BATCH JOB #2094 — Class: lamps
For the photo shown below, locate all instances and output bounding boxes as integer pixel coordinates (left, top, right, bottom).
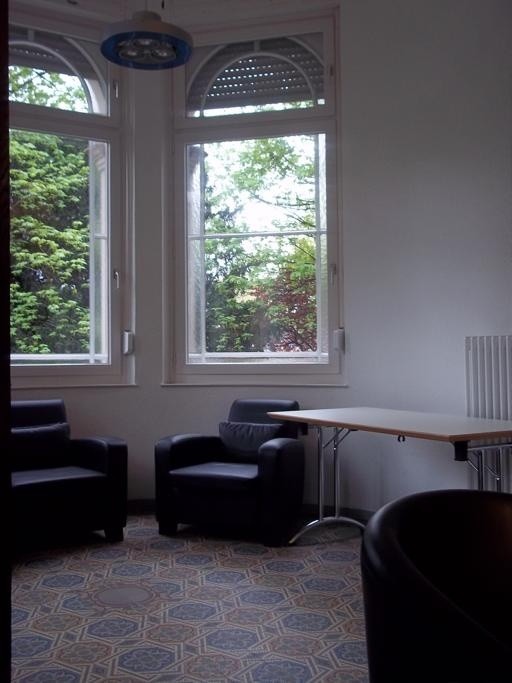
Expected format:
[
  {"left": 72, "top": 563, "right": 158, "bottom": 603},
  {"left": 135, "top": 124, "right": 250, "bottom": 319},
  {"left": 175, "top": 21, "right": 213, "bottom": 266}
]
[{"left": 100, "top": 2, "right": 193, "bottom": 70}]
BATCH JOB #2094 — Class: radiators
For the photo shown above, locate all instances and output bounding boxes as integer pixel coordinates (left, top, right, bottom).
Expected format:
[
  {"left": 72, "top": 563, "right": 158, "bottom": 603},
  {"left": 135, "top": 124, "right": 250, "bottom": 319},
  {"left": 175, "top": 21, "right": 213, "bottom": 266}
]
[{"left": 464, "top": 336, "right": 512, "bottom": 492}]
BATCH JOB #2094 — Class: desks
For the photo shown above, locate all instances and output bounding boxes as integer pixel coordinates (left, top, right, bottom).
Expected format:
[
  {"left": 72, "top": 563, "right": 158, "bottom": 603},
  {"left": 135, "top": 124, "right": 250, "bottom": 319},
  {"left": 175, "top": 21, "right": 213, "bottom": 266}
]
[{"left": 264, "top": 407, "right": 512, "bottom": 544}]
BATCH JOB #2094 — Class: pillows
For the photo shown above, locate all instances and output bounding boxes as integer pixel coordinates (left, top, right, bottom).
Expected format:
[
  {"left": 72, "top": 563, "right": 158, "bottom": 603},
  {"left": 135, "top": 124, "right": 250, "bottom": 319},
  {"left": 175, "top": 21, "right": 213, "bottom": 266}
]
[
  {"left": 10, "top": 421, "right": 70, "bottom": 468},
  {"left": 218, "top": 421, "right": 283, "bottom": 464}
]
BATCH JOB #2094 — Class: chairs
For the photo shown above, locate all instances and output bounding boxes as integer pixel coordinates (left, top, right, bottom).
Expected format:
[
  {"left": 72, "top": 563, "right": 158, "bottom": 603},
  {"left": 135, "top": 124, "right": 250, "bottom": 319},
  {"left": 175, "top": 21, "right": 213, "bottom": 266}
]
[
  {"left": 361, "top": 489, "right": 512, "bottom": 683},
  {"left": 154, "top": 398, "right": 306, "bottom": 548},
  {"left": 9, "top": 399, "right": 128, "bottom": 563}
]
